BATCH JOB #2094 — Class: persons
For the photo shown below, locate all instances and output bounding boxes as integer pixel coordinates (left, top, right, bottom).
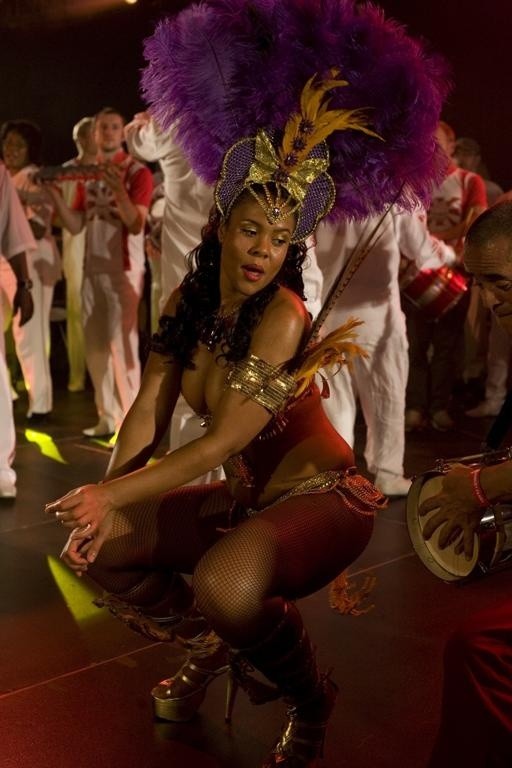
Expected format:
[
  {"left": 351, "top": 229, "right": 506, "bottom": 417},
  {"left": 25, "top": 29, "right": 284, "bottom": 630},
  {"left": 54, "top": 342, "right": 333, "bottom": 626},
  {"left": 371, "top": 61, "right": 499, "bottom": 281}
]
[
  {"left": 425, "top": 194, "right": 512, "bottom": 767},
  {"left": 1, "top": 100, "right": 512, "bottom": 511},
  {"left": 40, "top": 172, "right": 393, "bottom": 768}
]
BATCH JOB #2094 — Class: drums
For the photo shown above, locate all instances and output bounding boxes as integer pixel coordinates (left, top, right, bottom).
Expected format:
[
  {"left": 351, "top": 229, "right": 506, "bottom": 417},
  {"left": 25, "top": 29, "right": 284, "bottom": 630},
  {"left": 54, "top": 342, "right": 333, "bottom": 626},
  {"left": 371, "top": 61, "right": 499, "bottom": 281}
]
[
  {"left": 405, "top": 443, "right": 509, "bottom": 583},
  {"left": 395, "top": 239, "right": 470, "bottom": 324}
]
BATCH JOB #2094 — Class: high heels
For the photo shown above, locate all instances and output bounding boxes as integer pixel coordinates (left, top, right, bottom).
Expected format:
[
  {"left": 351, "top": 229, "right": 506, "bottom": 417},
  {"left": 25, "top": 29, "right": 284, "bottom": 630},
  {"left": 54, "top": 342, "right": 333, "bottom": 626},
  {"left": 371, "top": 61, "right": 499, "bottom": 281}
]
[
  {"left": 151, "top": 644, "right": 237, "bottom": 722},
  {"left": 272, "top": 682, "right": 329, "bottom": 764}
]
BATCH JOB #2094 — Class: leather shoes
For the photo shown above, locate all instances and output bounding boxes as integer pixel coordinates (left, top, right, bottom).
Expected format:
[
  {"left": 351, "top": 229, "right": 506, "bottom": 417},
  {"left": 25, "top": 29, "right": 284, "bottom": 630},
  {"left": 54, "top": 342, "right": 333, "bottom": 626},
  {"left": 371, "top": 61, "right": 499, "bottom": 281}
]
[
  {"left": 69, "top": 380, "right": 85, "bottom": 391},
  {"left": 27, "top": 402, "right": 47, "bottom": 417},
  {"left": 83, "top": 422, "right": 114, "bottom": 436},
  {"left": 375, "top": 476, "right": 412, "bottom": 495},
  {"left": 1, "top": 485, "right": 18, "bottom": 498}
]
